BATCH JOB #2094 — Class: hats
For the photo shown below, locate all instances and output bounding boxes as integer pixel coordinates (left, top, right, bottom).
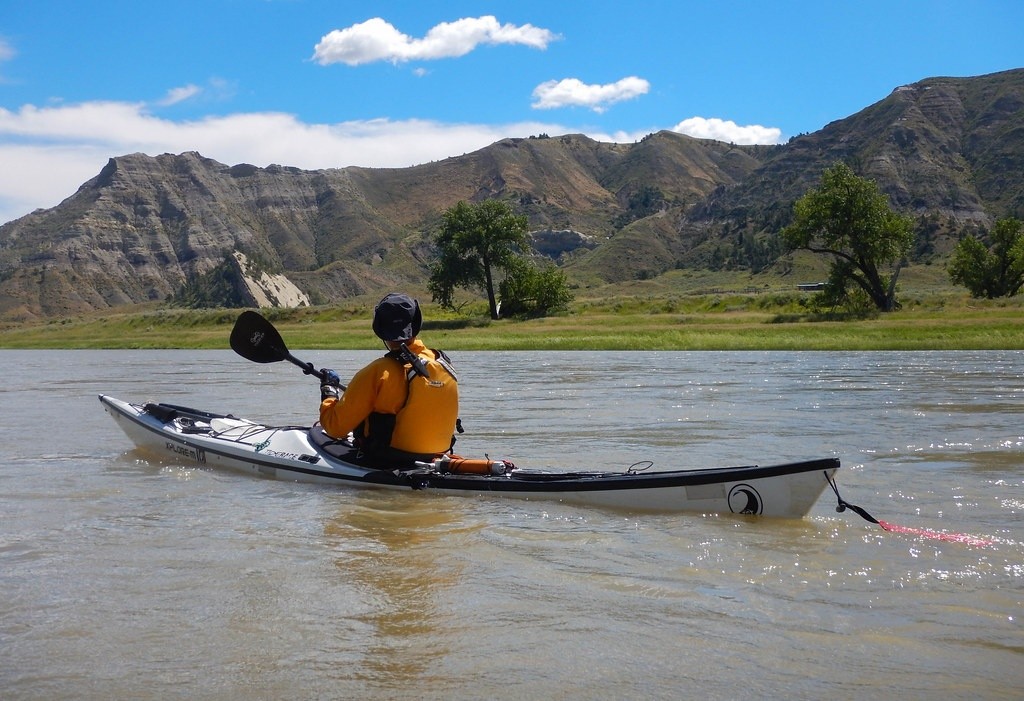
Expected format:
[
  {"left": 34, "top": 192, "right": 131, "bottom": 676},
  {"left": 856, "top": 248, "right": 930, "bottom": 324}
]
[{"left": 372, "top": 293, "right": 422, "bottom": 341}]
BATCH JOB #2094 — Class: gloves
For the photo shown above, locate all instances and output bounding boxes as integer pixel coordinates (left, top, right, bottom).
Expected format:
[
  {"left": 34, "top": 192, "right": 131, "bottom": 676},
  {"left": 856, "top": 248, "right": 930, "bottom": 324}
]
[{"left": 318, "top": 368, "right": 340, "bottom": 394}]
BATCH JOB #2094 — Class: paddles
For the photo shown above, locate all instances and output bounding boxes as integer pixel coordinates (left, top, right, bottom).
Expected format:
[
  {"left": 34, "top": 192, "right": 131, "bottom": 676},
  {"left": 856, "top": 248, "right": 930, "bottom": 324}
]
[{"left": 228, "top": 310, "right": 347, "bottom": 392}]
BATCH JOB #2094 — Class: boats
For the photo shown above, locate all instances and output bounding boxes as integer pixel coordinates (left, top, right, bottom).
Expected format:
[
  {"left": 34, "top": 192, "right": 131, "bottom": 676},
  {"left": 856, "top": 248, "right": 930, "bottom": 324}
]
[{"left": 97, "top": 391, "right": 840, "bottom": 522}]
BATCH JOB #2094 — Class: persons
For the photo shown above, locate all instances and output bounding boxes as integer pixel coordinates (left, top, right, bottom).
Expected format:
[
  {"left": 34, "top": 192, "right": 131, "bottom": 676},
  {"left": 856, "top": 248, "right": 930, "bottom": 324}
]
[{"left": 319, "top": 292, "right": 459, "bottom": 470}]
